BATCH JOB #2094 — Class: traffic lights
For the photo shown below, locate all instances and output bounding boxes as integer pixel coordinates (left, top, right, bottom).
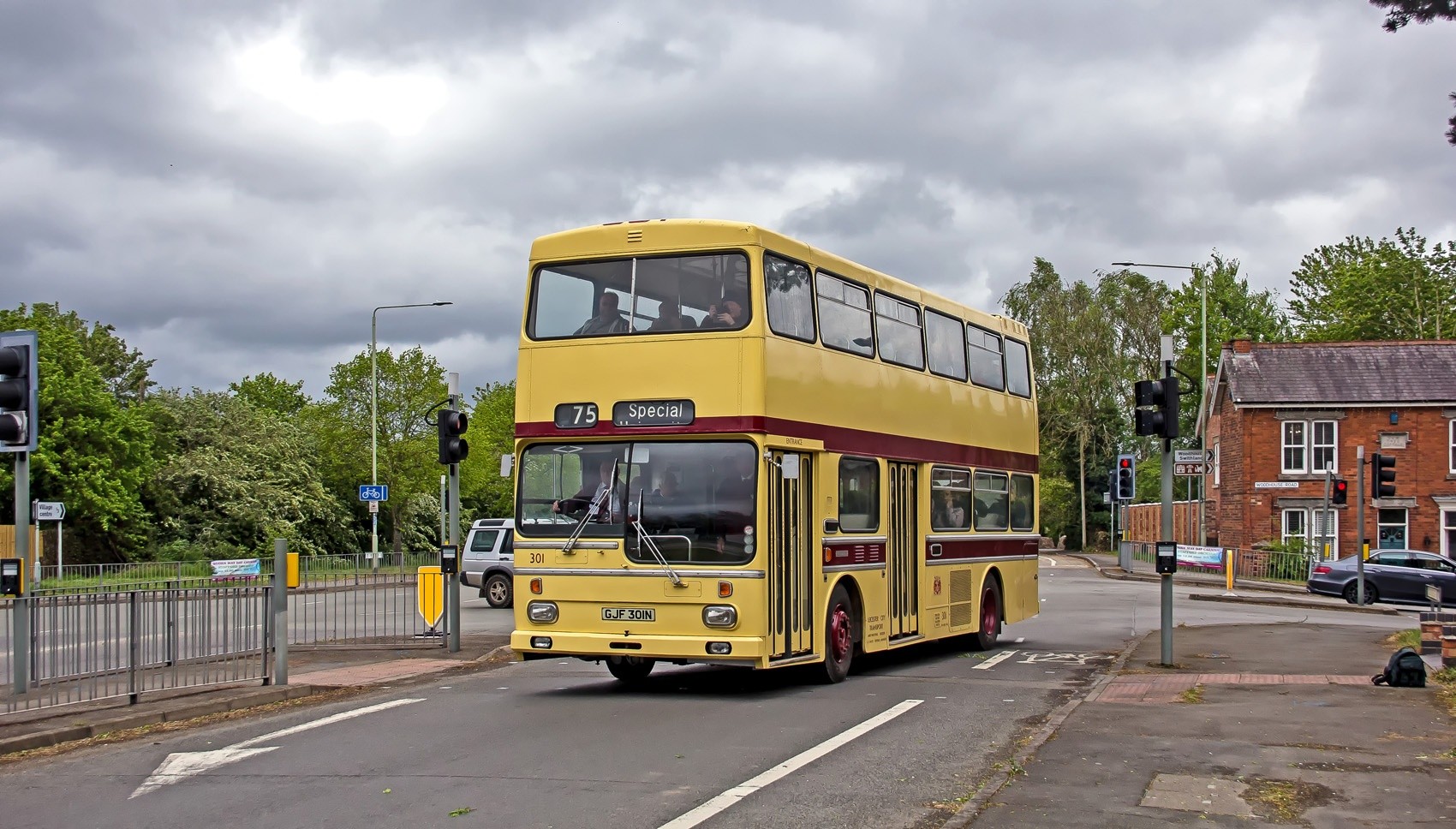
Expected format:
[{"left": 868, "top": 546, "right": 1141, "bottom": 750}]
[
  {"left": 1118, "top": 454, "right": 1135, "bottom": 499},
  {"left": 438, "top": 408, "right": 469, "bottom": 466},
  {"left": 1333, "top": 479, "right": 1347, "bottom": 504},
  {"left": 0, "top": 330, "right": 38, "bottom": 453},
  {"left": 1371, "top": 453, "right": 1396, "bottom": 500}
]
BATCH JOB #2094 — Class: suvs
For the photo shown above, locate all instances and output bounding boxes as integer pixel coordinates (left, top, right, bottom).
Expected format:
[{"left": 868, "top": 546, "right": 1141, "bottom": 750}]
[{"left": 459, "top": 518, "right": 572, "bottom": 609}]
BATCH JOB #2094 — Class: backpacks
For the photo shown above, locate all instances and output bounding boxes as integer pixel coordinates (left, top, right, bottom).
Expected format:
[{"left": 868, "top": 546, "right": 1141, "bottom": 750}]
[{"left": 1372, "top": 646, "right": 1428, "bottom": 688}]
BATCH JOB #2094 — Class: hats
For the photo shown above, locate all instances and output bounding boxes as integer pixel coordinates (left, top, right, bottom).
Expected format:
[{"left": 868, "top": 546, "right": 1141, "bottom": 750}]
[{"left": 722, "top": 289, "right": 745, "bottom": 309}]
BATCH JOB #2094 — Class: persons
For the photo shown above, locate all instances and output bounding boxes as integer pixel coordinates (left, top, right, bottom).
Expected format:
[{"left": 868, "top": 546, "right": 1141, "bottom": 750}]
[
  {"left": 931, "top": 479, "right": 1027, "bottom": 528},
  {"left": 629, "top": 472, "right": 700, "bottom": 529},
  {"left": 553, "top": 458, "right": 627, "bottom": 524},
  {"left": 714, "top": 451, "right": 755, "bottom": 554},
  {"left": 572, "top": 290, "right": 750, "bottom": 337}
]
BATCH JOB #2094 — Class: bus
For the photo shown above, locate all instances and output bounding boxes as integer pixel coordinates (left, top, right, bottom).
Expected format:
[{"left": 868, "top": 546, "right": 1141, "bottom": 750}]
[{"left": 498, "top": 217, "right": 1038, "bottom": 687}]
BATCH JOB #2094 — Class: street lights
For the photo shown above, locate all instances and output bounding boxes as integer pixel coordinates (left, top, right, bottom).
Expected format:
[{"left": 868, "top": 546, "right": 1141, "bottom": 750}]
[
  {"left": 370, "top": 301, "right": 454, "bottom": 575},
  {"left": 1112, "top": 260, "right": 1207, "bottom": 547}
]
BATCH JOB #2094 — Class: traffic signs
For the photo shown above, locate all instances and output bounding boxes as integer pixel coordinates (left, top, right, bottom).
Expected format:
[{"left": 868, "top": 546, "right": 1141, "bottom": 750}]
[
  {"left": 1174, "top": 462, "right": 1214, "bottom": 475},
  {"left": 32, "top": 500, "right": 66, "bottom": 520},
  {"left": 1174, "top": 449, "right": 1215, "bottom": 463}
]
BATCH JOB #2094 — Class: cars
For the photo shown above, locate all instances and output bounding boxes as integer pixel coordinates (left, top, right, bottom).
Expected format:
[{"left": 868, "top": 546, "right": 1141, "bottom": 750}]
[{"left": 1306, "top": 548, "right": 1456, "bottom": 609}]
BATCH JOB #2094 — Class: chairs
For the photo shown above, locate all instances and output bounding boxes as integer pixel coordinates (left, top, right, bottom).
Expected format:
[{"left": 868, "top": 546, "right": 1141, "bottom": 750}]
[{"left": 781, "top": 322, "right": 1017, "bottom": 393}]
